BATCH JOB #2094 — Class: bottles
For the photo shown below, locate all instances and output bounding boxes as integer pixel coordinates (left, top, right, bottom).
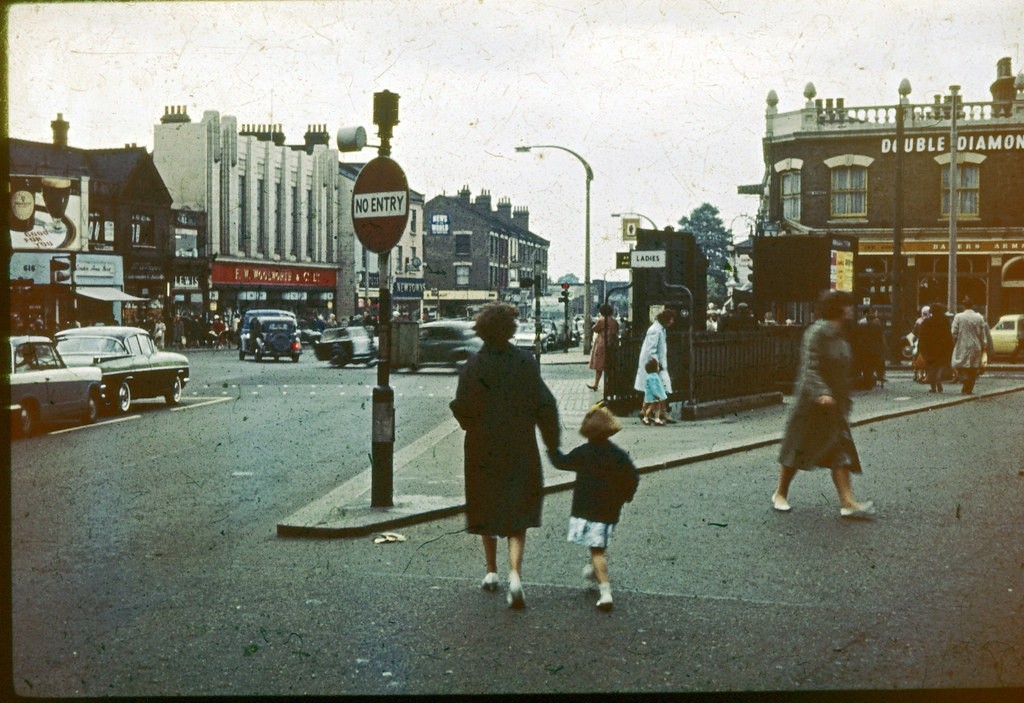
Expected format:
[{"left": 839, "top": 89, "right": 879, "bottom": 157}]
[
  {"left": 8, "top": 177, "right": 36, "bottom": 231},
  {"left": 982, "top": 346, "right": 987, "bottom": 368}
]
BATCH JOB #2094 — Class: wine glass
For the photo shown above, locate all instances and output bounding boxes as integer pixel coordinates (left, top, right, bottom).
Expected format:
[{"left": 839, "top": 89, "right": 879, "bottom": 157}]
[{"left": 41, "top": 178, "right": 71, "bottom": 233}]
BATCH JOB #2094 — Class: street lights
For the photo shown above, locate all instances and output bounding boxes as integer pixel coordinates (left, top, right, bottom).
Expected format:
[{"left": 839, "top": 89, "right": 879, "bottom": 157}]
[
  {"left": 515, "top": 144, "right": 594, "bottom": 355},
  {"left": 611, "top": 212, "right": 658, "bottom": 229}
]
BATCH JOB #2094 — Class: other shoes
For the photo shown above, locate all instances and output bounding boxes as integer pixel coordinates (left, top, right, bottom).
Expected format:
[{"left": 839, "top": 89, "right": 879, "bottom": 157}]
[
  {"left": 913, "top": 374, "right": 929, "bottom": 384},
  {"left": 482, "top": 572, "right": 500, "bottom": 591},
  {"left": 950, "top": 378, "right": 960, "bottom": 383},
  {"left": 582, "top": 564, "right": 596, "bottom": 579},
  {"left": 961, "top": 389, "right": 975, "bottom": 395},
  {"left": 840, "top": 501, "right": 874, "bottom": 516},
  {"left": 938, "top": 387, "right": 943, "bottom": 392},
  {"left": 595, "top": 592, "right": 613, "bottom": 608},
  {"left": 587, "top": 384, "right": 598, "bottom": 391},
  {"left": 772, "top": 493, "right": 792, "bottom": 512},
  {"left": 639, "top": 410, "right": 678, "bottom": 427},
  {"left": 929, "top": 389, "right": 936, "bottom": 393},
  {"left": 506, "top": 577, "right": 527, "bottom": 608}
]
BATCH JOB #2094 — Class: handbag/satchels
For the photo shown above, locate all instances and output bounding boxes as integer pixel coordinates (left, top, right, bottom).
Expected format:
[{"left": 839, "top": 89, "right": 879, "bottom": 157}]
[{"left": 913, "top": 353, "right": 927, "bottom": 369}]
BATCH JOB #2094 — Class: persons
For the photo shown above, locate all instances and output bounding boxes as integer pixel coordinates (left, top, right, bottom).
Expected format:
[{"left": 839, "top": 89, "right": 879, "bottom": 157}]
[
  {"left": 706, "top": 302, "right": 776, "bottom": 331},
  {"left": 632, "top": 310, "right": 677, "bottom": 424},
  {"left": 391, "top": 308, "right": 430, "bottom": 337},
  {"left": 546, "top": 406, "right": 638, "bottom": 605},
  {"left": 586, "top": 303, "right": 619, "bottom": 391},
  {"left": 859, "top": 298, "right": 993, "bottom": 394},
  {"left": 182, "top": 311, "right": 240, "bottom": 349},
  {"left": 773, "top": 291, "right": 878, "bottom": 517},
  {"left": 448, "top": 299, "right": 562, "bottom": 604},
  {"left": 16, "top": 308, "right": 186, "bottom": 351},
  {"left": 316, "top": 307, "right": 379, "bottom": 336}
]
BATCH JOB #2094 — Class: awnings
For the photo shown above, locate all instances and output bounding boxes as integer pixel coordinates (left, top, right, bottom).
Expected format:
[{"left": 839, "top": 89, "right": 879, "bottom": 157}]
[{"left": 70, "top": 285, "right": 151, "bottom": 301}]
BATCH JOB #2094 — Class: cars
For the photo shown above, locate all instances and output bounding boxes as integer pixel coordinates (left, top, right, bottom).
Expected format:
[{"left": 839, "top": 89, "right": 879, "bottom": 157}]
[
  {"left": 237, "top": 309, "right": 304, "bottom": 363},
  {"left": 852, "top": 302, "right": 1024, "bottom": 360},
  {"left": 32, "top": 325, "right": 192, "bottom": 419},
  {"left": 296, "top": 313, "right": 601, "bottom": 372},
  {"left": 7, "top": 334, "right": 103, "bottom": 441}
]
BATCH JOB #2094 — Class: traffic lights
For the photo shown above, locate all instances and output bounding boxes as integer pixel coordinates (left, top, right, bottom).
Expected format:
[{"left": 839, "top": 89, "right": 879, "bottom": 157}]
[{"left": 560, "top": 282, "right": 569, "bottom": 296}]
